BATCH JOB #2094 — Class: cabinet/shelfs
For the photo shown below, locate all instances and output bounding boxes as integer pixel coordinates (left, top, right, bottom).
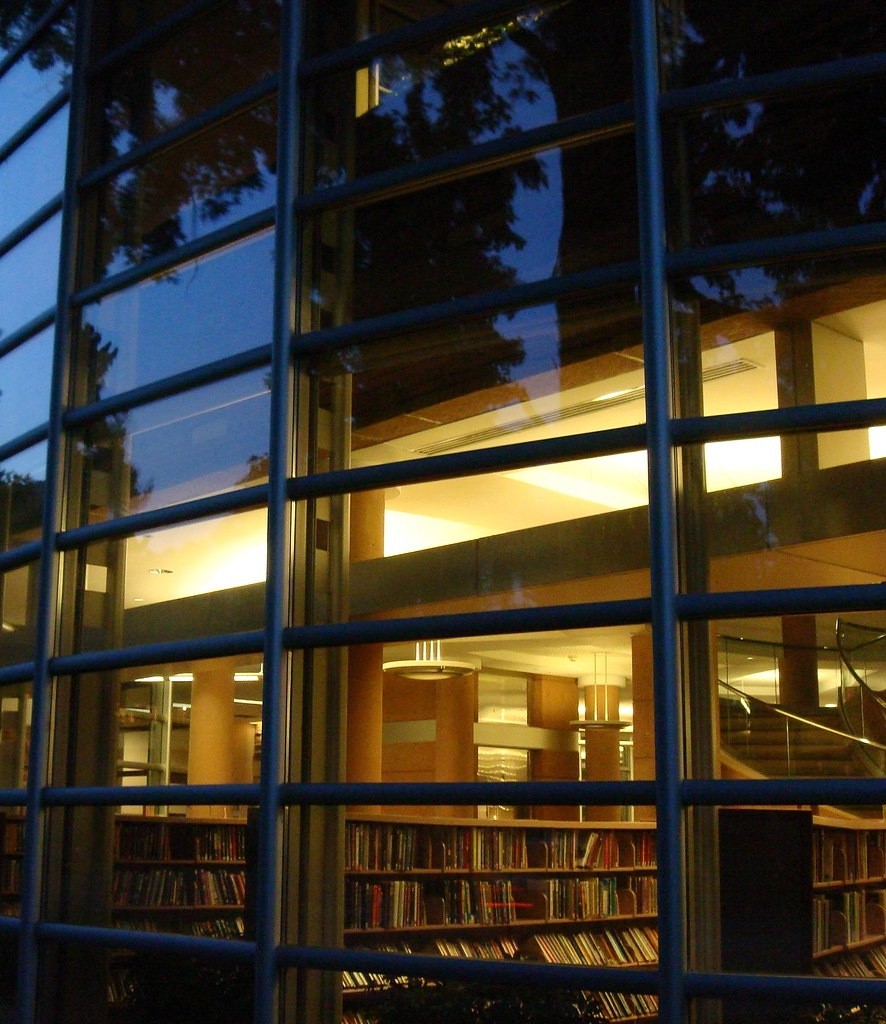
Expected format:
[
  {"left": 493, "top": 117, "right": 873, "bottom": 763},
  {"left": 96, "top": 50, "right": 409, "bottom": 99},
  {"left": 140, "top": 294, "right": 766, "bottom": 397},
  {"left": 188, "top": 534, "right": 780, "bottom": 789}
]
[{"left": 1, "top": 811, "right": 886, "bottom": 1024}]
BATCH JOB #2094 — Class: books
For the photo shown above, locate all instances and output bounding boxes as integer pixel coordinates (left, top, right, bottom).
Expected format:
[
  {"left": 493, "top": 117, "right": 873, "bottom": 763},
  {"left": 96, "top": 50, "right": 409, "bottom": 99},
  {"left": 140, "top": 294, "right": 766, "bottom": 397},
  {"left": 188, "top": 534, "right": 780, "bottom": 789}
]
[
  {"left": 343, "top": 820, "right": 660, "bottom": 1024},
  {"left": 0, "top": 821, "right": 27, "bottom": 918},
  {"left": 105, "top": 822, "right": 248, "bottom": 1003},
  {"left": 810, "top": 828, "right": 886, "bottom": 980}
]
